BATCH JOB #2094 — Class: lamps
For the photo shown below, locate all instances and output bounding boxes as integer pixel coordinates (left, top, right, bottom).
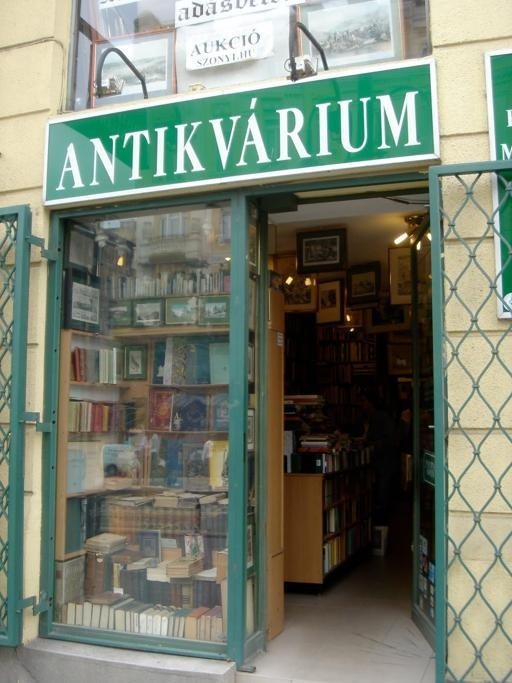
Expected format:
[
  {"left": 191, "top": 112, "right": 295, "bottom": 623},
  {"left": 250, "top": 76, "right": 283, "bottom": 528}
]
[
  {"left": 392, "top": 210, "right": 434, "bottom": 251},
  {"left": 280, "top": 18, "right": 333, "bottom": 82},
  {"left": 90, "top": 42, "right": 154, "bottom": 105}
]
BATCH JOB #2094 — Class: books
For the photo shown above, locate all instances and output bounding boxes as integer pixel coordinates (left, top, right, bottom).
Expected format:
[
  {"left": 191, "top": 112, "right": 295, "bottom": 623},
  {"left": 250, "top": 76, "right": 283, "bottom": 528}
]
[
  {"left": 284, "top": 329, "right": 430, "bottom": 573},
  {"left": 61, "top": 207, "right": 254, "bottom": 385},
  {"left": 67, "top": 388, "right": 253, "bottom": 641}
]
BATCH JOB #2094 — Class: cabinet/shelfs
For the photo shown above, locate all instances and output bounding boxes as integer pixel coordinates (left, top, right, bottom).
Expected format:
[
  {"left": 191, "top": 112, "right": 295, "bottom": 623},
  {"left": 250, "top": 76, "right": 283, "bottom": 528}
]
[
  {"left": 283, "top": 467, "right": 372, "bottom": 595},
  {"left": 52, "top": 289, "right": 236, "bottom": 564}
]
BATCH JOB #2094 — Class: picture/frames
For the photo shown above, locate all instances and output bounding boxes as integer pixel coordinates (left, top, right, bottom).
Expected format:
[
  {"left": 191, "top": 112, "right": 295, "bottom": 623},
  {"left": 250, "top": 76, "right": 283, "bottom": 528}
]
[
  {"left": 62, "top": 220, "right": 232, "bottom": 382},
  {"left": 271, "top": 223, "right": 431, "bottom": 324}
]
